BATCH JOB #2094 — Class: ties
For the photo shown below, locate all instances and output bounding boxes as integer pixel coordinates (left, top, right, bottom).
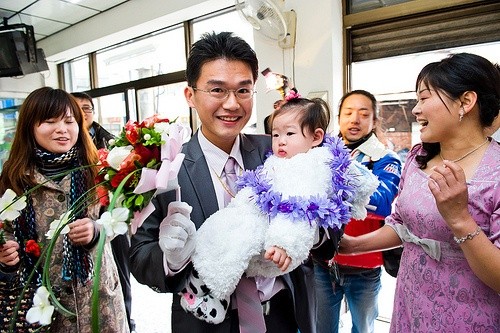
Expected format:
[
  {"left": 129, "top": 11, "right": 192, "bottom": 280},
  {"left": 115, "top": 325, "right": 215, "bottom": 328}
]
[{"left": 225, "top": 157, "right": 267, "bottom": 333}]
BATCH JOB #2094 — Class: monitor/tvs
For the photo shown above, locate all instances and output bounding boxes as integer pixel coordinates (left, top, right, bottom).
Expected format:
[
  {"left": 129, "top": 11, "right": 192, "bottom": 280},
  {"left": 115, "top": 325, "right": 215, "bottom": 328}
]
[{"left": 0, "top": 29, "right": 49, "bottom": 79}]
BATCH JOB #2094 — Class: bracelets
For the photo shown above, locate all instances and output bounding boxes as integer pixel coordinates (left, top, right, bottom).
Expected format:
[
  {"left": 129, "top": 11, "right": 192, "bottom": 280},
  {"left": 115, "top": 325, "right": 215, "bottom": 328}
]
[{"left": 453, "top": 224, "right": 483, "bottom": 245}]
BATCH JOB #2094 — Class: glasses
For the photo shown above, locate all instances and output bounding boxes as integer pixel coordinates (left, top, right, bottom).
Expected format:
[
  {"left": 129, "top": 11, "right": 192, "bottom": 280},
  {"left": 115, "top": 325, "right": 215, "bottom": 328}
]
[
  {"left": 192, "top": 86, "right": 257, "bottom": 100},
  {"left": 81, "top": 106, "right": 94, "bottom": 113}
]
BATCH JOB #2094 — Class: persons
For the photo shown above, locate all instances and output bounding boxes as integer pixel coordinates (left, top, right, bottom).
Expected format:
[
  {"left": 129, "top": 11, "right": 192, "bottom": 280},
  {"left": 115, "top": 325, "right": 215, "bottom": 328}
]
[
  {"left": 334, "top": 54, "right": 500, "bottom": 332},
  {"left": 72, "top": 91, "right": 143, "bottom": 332},
  {"left": 0, "top": 88, "right": 128, "bottom": 333},
  {"left": 128, "top": 30, "right": 347, "bottom": 332},
  {"left": 312, "top": 89, "right": 402, "bottom": 333},
  {"left": 178, "top": 88, "right": 336, "bottom": 324}
]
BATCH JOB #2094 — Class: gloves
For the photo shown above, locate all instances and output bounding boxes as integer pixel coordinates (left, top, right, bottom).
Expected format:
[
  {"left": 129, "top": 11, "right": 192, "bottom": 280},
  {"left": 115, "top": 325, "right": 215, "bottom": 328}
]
[{"left": 158, "top": 201, "right": 197, "bottom": 270}]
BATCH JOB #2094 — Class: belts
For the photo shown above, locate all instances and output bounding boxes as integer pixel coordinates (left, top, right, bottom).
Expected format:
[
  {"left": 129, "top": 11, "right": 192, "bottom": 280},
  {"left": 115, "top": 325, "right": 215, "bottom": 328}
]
[{"left": 231, "top": 288, "right": 292, "bottom": 321}]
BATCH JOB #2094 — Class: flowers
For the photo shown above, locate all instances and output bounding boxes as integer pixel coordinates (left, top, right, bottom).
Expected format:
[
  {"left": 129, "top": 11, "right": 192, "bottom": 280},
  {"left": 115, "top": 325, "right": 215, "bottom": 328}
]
[{"left": 1, "top": 112, "right": 192, "bottom": 332}]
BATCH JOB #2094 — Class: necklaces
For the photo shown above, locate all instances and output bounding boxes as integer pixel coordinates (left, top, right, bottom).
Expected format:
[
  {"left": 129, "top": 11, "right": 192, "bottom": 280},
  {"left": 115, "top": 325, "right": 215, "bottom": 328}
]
[{"left": 439, "top": 136, "right": 491, "bottom": 172}]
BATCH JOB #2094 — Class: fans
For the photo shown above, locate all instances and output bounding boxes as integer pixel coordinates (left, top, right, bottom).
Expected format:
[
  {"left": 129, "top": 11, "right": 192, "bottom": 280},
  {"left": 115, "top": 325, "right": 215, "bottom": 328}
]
[{"left": 233, "top": 0, "right": 299, "bottom": 47}]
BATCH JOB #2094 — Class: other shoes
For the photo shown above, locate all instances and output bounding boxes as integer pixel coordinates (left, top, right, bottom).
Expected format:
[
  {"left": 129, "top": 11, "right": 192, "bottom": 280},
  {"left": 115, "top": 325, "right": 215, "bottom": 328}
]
[
  {"left": 180, "top": 290, "right": 230, "bottom": 324},
  {"left": 175, "top": 272, "right": 210, "bottom": 297}
]
